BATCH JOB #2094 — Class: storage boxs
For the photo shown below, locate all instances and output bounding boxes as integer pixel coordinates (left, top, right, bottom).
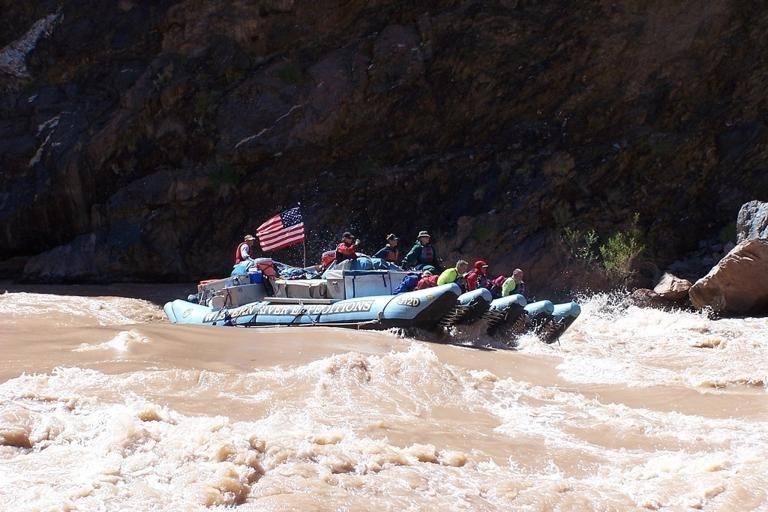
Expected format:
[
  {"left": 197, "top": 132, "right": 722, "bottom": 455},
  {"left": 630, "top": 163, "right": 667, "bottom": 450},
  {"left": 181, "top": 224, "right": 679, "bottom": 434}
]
[
  {"left": 197, "top": 271, "right": 267, "bottom": 309},
  {"left": 275, "top": 270, "right": 345, "bottom": 300}
]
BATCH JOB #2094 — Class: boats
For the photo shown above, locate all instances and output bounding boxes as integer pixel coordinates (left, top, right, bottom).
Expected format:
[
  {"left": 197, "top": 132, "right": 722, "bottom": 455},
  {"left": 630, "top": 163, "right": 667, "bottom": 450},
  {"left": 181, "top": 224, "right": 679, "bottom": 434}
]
[{"left": 163, "top": 282, "right": 583, "bottom": 345}]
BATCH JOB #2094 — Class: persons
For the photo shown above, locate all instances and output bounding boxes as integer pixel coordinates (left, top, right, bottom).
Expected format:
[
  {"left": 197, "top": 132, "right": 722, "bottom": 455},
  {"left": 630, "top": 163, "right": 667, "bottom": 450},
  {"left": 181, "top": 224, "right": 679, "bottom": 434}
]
[
  {"left": 372, "top": 233, "right": 401, "bottom": 262},
  {"left": 336, "top": 232, "right": 360, "bottom": 264},
  {"left": 393, "top": 260, "right": 525, "bottom": 300},
  {"left": 235, "top": 235, "right": 256, "bottom": 264},
  {"left": 401, "top": 231, "right": 436, "bottom": 268}
]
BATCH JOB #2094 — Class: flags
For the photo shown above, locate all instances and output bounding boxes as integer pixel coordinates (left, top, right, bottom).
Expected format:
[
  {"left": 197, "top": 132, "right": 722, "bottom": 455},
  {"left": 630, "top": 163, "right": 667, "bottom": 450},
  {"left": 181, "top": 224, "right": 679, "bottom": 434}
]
[{"left": 256, "top": 206, "right": 305, "bottom": 253}]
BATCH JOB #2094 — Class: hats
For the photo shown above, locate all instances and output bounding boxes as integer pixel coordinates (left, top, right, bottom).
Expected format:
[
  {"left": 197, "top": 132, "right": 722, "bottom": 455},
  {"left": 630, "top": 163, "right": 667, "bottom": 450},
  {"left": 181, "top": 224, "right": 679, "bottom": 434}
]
[
  {"left": 418, "top": 231, "right": 431, "bottom": 238},
  {"left": 342, "top": 231, "right": 354, "bottom": 238},
  {"left": 386, "top": 234, "right": 398, "bottom": 241},
  {"left": 244, "top": 234, "right": 256, "bottom": 242}
]
